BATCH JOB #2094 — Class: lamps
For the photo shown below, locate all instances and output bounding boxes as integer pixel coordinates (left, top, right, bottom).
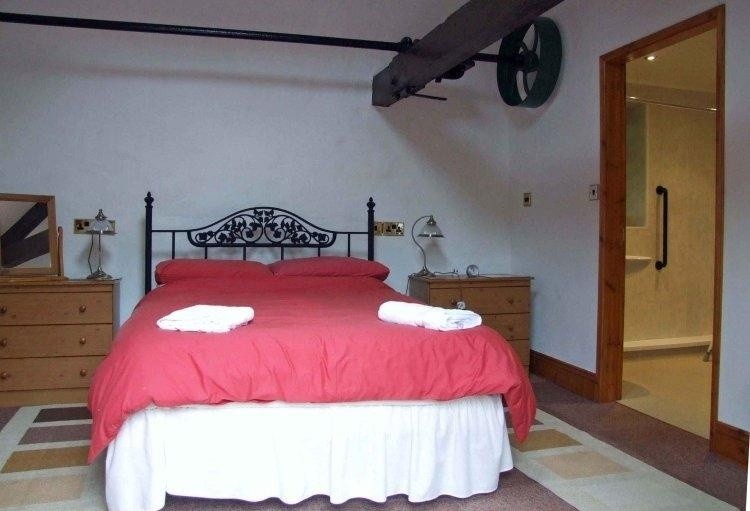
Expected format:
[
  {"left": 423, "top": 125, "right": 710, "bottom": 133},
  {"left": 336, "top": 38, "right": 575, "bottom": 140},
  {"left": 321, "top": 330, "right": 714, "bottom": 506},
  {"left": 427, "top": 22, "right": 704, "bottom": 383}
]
[
  {"left": 410, "top": 215, "right": 444, "bottom": 277},
  {"left": 88, "top": 207, "right": 113, "bottom": 279}
]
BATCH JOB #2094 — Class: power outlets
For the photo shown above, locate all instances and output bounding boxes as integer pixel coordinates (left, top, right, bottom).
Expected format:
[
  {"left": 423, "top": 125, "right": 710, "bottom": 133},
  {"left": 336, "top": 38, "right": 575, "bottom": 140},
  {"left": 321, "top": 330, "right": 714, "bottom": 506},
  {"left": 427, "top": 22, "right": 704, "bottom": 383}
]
[
  {"left": 74, "top": 219, "right": 94, "bottom": 235},
  {"left": 375, "top": 221, "right": 404, "bottom": 237}
]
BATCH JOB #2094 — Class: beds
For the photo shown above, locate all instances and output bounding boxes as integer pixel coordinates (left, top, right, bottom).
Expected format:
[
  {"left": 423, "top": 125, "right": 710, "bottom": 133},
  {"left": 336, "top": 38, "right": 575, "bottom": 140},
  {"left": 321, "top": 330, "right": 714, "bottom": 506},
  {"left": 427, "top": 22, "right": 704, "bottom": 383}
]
[{"left": 84, "top": 190, "right": 536, "bottom": 511}]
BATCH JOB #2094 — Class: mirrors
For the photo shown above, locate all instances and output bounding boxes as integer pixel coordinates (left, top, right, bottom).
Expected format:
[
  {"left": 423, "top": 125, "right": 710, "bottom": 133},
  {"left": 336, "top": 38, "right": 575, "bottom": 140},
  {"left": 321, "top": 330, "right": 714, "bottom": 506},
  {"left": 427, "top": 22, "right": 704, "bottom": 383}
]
[{"left": 0, "top": 194, "right": 59, "bottom": 277}]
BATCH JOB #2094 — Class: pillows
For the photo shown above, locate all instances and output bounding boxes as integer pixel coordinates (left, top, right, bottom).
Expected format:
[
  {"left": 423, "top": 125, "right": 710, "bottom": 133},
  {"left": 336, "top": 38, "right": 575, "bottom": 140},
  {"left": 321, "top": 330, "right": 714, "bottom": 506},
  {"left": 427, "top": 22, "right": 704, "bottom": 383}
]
[
  {"left": 273, "top": 255, "right": 388, "bottom": 277},
  {"left": 155, "top": 259, "right": 273, "bottom": 284}
]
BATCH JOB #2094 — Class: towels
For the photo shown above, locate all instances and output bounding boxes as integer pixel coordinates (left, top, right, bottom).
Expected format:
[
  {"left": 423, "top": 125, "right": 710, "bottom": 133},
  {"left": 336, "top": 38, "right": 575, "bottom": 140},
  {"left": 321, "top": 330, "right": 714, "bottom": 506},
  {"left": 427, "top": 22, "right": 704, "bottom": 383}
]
[
  {"left": 156, "top": 304, "right": 254, "bottom": 333},
  {"left": 378, "top": 300, "right": 483, "bottom": 332}
]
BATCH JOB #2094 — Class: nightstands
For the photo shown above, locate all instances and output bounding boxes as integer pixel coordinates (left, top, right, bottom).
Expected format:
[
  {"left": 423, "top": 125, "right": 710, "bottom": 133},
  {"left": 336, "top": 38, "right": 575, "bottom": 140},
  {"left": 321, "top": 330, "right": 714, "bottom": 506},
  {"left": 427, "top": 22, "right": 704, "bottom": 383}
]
[
  {"left": 0, "top": 278, "right": 120, "bottom": 406},
  {"left": 411, "top": 275, "right": 534, "bottom": 378}
]
